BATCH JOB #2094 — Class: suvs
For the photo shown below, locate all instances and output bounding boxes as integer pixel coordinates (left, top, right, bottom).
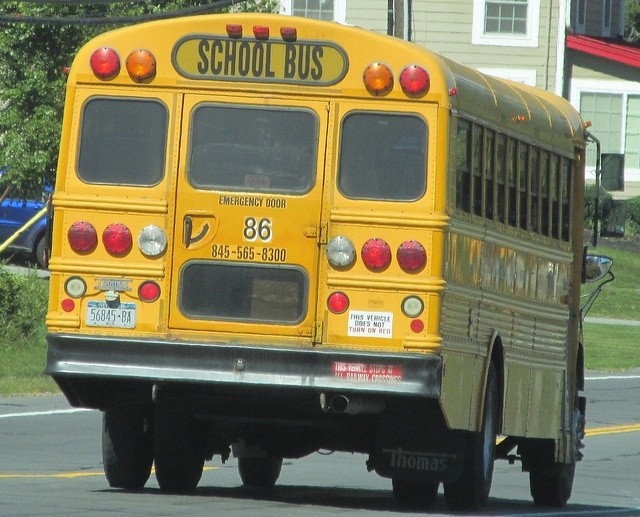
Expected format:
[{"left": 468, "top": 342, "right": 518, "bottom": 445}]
[{"left": 0, "top": 163, "right": 54, "bottom": 271}]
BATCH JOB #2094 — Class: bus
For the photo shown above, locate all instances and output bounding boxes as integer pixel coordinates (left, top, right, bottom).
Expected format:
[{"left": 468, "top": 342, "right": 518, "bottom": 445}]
[{"left": 40, "top": 12, "right": 627, "bottom": 511}]
